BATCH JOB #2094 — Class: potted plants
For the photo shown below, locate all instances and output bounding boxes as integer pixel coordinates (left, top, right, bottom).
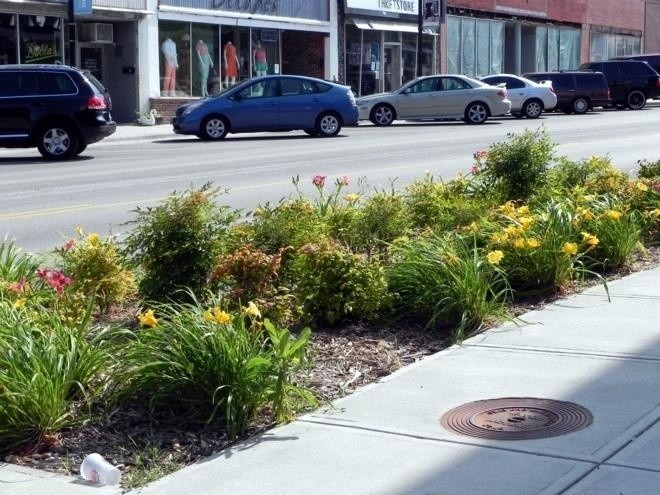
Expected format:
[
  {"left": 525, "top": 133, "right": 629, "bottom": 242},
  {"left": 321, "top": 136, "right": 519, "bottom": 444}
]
[{"left": 135, "top": 111, "right": 163, "bottom": 124}]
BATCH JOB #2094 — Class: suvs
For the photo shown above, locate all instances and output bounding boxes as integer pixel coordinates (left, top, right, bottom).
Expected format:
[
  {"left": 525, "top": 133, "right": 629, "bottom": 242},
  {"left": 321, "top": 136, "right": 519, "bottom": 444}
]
[
  {"left": 0, "top": 62, "right": 118, "bottom": 163},
  {"left": 522, "top": 56, "right": 660, "bottom": 114}
]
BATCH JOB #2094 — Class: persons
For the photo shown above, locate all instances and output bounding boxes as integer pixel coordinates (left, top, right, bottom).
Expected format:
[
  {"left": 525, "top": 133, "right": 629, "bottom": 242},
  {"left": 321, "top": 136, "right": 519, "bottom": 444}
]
[
  {"left": 253, "top": 44, "right": 268, "bottom": 89},
  {"left": 224, "top": 41, "right": 240, "bottom": 88},
  {"left": 162, "top": 38, "right": 178, "bottom": 97},
  {"left": 196, "top": 40, "right": 213, "bottom": 97}
]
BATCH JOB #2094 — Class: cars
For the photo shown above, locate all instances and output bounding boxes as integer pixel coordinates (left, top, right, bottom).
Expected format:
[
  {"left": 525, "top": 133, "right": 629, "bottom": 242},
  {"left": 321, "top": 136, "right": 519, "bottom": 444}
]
[
  {"left": 171, "top": 73, "right": 361, "bottom": 140},
  {"left": 353, "top": 73, "right": 513, "bottom": 127},
  {"left": 477, "top": 73, "right": 558, "bottom": 119}
]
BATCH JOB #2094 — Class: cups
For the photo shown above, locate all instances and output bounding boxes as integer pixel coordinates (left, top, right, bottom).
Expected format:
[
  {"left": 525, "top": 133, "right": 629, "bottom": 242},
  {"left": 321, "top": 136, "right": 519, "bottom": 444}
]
[{"left": 80, "top": 452, "right": 122, "bottom": 485}]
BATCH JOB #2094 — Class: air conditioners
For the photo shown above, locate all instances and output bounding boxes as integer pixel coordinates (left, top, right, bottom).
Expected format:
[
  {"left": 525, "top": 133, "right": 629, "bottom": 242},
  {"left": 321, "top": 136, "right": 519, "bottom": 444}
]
[{"left": 81, "top": 22, "right": 113, "bottom": 45}]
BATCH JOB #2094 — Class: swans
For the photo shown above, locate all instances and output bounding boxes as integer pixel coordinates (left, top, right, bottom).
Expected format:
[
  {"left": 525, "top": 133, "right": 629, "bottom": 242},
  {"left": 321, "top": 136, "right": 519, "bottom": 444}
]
[{"left": 136, "top": 109, "right": 158, "bottom": 126}]
